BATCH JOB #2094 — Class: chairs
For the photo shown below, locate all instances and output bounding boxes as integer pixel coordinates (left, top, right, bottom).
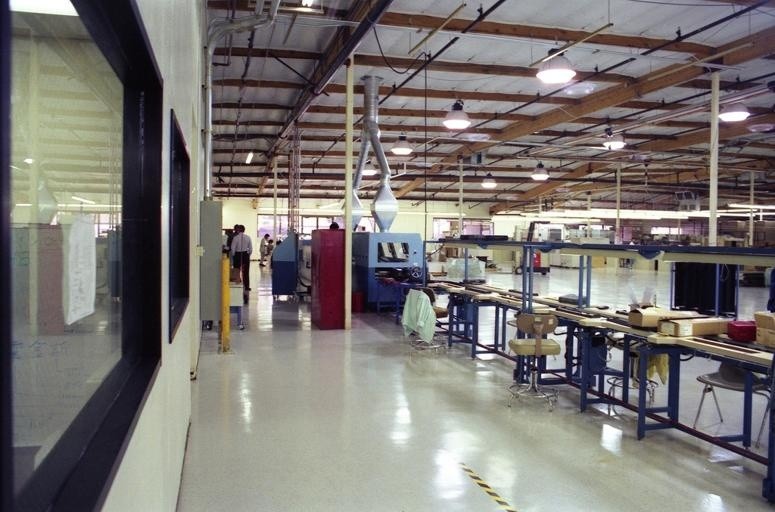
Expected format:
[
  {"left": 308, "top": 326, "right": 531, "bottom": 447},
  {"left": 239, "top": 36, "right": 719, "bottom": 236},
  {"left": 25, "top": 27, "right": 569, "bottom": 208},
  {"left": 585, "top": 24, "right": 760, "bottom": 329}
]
[
  {"left": 504, "top": 311, "right": 563, "bottom": 414},
  {"left": 604, "top": 334, "right": 662, "bottom": 421},
  {"left": 397, "top": 286, "right": 450, "bottom": 351},
  {"left": 691, "top": 347, "right": 772, "bottom": 447}
]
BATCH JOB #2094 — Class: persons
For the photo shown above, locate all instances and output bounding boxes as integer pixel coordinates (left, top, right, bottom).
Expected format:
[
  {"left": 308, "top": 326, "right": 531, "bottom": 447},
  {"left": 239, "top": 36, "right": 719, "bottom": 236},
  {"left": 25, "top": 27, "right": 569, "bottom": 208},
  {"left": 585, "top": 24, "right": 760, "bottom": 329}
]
[
  {"left": 226, "top": 224, "right": 238, "bottom": 250},
  {"left": 230, "top": 225, "right": 253, "bottom": 291},
  {"left": 259, "top": 233, "right": 282, "bottom": 267},
  {"left": 329, "top": 223, "right": 339, "bottom": 229}
]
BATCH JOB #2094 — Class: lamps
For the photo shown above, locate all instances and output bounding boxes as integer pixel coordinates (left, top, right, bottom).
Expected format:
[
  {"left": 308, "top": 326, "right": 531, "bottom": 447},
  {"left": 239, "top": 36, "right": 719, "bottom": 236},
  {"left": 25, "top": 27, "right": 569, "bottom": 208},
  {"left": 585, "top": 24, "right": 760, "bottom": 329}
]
[
  {"left": 602, "top": 126, "right": 627, "bottom": 150},
  {"left": 535, "top": 33, "right": 578, "bottom": 86},
  {"left": 718, "top": 92, "right": 750, "bottom": 123},
  {"left": 479, "top": 167, "right": 500, "bottom": 190},
  {"left": 441, "top": 87, "right": 472, "bottom": 132},
  {"left": 389, "top": 121, "right": 414, "bottom": 157},
  {"left": 361, "top": 155, "right": 378, "bottom": 177},
  {"left": 529, "top": 158, "right": 550, "bottom": 183}
]
